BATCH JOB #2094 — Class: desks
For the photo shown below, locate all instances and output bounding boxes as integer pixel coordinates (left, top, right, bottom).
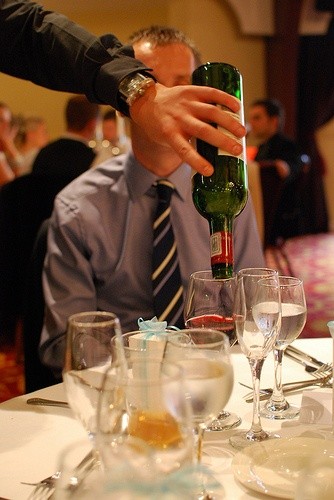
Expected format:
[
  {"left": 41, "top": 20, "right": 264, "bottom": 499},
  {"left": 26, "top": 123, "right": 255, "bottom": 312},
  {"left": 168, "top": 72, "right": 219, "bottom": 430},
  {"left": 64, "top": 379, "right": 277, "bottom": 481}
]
[{"left": 0, "top": 338, "right": 334, "bottom": 500}]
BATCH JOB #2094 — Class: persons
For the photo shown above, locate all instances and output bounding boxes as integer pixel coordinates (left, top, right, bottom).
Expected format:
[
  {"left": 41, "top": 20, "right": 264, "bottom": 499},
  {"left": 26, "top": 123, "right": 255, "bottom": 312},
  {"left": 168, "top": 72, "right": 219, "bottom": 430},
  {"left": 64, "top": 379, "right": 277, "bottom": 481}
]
[{"left": 0, "top": 0, "right": 310, "bottom": 377}]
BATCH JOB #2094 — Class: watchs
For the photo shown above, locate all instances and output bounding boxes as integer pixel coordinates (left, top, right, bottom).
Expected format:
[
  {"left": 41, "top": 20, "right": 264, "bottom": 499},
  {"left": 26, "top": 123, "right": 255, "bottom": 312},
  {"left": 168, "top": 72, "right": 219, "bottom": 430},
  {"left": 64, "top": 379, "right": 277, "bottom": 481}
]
[{"left": 117, "top": 70, "right": 156, "bottom": 119}]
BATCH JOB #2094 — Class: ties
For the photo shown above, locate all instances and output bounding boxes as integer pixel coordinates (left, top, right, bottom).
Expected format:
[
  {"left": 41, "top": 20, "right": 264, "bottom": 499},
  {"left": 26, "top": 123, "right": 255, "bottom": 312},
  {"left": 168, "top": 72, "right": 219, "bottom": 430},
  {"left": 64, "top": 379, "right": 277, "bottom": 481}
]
[{"left": 151, "top": 179, "right": 186, "bottom": 331}]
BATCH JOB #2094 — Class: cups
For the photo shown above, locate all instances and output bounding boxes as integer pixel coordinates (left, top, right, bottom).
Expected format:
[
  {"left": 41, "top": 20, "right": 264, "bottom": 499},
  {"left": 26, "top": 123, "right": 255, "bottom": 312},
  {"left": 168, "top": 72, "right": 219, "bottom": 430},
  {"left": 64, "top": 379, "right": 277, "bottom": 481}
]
[{"left": 109, "top": 329, "right": 197, "bottom": 474}]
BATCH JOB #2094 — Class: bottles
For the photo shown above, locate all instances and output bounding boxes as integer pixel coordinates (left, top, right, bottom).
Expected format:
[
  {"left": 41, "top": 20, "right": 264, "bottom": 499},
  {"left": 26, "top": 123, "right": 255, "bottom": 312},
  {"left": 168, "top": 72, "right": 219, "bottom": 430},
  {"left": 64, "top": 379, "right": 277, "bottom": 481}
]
[{"left": 190, "top": 61, "right": 249, "bottom": 280}]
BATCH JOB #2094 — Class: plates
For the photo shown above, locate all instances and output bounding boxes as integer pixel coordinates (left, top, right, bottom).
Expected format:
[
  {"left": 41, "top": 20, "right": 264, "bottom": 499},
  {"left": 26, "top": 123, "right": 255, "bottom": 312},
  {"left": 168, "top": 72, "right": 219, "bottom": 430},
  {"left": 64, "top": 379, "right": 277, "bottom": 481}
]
[{"left": 232, "top": 436, "right": 334, "bottom": 500}]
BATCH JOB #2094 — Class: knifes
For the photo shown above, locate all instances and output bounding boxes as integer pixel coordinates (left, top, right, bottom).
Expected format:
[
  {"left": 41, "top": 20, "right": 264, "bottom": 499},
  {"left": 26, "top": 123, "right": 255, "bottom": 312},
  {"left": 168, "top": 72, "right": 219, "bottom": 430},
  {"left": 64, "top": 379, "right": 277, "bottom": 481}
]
[{"left": 277, "top": 341, "right": 333, "bottom": 374}]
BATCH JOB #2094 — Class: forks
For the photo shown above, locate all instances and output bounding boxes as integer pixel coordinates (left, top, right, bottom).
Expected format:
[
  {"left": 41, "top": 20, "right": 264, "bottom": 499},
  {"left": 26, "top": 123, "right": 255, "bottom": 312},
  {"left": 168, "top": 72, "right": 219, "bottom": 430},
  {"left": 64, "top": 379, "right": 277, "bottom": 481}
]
[
  {"left": 239, "top": 373, "right": 331, "bottom": 396},
  {"left": 19, "top": 447, "right": 98, "bottom": 500},
  {"left": 284, "top": 352, "right": 333, "bottom": 375}
]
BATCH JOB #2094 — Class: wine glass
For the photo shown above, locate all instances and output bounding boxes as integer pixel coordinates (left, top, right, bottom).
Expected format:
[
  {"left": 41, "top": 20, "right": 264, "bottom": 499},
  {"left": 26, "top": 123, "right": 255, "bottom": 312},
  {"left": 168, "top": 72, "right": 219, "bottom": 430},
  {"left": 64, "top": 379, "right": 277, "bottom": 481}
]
[
  {"left": 62, "top": 311, "right": 127, "bottom": 476},
  {"left": 252, "top": 274, "right": 307, "bottom": 421},
  {"left": 229, "top": 266, "right": 283, "bottom": 451},
  {"left": 184, "top": 269, "right": 247, "bottom": 433},
  {"left": 160, "top": 328, "right": 234, "bottom": 500}
]
